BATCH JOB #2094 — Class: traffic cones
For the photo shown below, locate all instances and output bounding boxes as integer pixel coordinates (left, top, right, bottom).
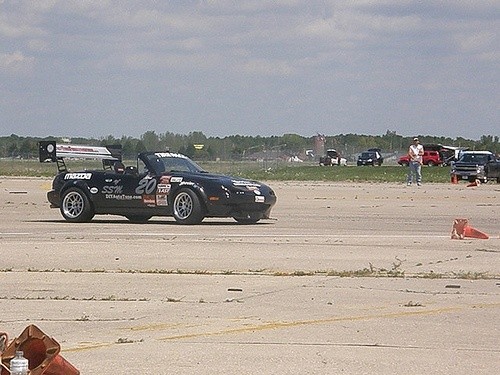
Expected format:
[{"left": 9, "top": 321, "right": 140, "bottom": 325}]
[
  {"left": 0, "top": 324, "right": 80, "bottom": 375},
  {"left": 467, "top": 179, "right": 480, "bottom": 187},
  {"left": 450, "top": 218, "right": 489, "bottom": 240}
]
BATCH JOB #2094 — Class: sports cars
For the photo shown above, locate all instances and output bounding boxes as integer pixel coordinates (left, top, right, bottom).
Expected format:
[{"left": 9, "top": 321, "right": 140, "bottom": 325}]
[{"left": 38, "top": 138, "right": 277, "bottom": 223}]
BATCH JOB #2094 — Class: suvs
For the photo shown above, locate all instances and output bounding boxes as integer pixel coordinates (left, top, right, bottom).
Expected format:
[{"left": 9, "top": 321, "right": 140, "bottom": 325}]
[
  {"left": 451, "top": 150, "right": 500, "bottom": 183},
  {"left": 398, "top": 151, "right": 441, "bottom": 167}
]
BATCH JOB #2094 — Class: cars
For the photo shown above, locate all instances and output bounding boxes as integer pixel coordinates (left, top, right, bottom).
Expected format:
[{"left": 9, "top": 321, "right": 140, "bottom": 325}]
[
  {"left": 319, "top": 149, "right": 347, "bottom": 167},
  {"left": 357, "top": 148, "right": 383, "bottom": 167},
  {"left": 443, "top": 155, "right": 460, "bottom": 167}
]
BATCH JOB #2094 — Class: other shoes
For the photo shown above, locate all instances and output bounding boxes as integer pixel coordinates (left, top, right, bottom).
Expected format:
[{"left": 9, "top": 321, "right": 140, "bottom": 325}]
[
  {"left": 417, "top": 182, "right": 422, "bottom": 186},
  {"left": 407, "top": 182, "right": 411, "bottom": 186}
]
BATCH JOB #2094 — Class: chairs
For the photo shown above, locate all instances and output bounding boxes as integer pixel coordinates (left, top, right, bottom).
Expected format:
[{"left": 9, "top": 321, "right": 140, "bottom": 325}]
[
  {"left": 126, "top": 166, "right": 137, "bottom": 176},
  {"left": 114, "top": 162, "right": 125, "bottom": 174}
]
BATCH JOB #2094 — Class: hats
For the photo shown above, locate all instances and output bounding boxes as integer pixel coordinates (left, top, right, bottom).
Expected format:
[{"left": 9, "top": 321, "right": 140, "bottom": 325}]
[{"left": 414, "top": 138, "right": 419, "bottom": 141}]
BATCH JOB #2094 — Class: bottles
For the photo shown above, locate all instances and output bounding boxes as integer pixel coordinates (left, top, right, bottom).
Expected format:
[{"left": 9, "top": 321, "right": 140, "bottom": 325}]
[{"left": 9, "top": 349, "right": 28, "bottom": 375}]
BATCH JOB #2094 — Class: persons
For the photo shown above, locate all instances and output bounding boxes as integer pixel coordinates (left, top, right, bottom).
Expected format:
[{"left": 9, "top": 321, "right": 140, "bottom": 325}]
[{"left": 407, "top": 138, "right": 424, "bottom": 186}]
